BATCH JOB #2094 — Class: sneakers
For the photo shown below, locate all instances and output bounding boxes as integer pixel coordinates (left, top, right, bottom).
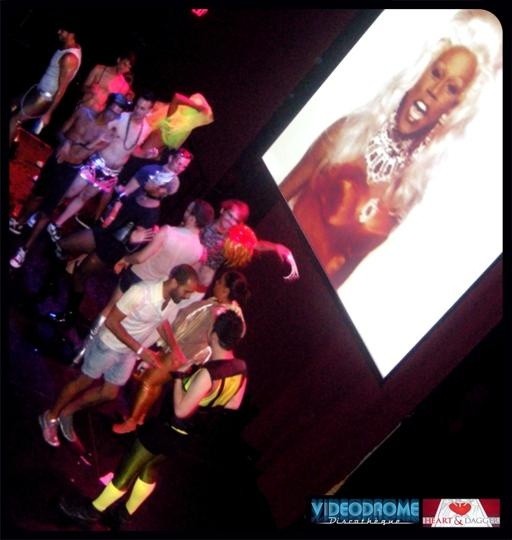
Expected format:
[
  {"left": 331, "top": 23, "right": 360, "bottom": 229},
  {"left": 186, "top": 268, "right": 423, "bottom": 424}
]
[
  {"left": 112, "top": 422, "right": 138, "bottom": 434},
  {"left": 38, "top": 409, "right": 60, "bottom": 447},
  {"left": 122, "top": 415, "right": 144, "bottom": 431},
  {"left": 58, "top": 410, "right": 77, "bottom": 442},
  {"left": 9, "top": 220, "right": 23, "bottom": 236},
  {"left": 10, "top": 247, "right": 28, "bottom": 269},
  {"left": 59, "top": 496, "right": 103, "bottom": 529},
  {"left": 27, "top": 213, "right": 37, "bottom": 228},
  {"left": 111, "top": 501, "right": 132, "bottom": 532},
  {"left": 46, "top": 223, "right": 61, "bottom": 243}
]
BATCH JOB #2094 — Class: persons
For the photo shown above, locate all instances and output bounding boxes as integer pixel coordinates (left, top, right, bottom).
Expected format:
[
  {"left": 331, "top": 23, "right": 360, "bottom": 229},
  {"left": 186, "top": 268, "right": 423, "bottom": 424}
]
[
  {"left": 273, "top": 29, "right": 485, "bottom": 291},
  {"left": 5, "top": 16, "right": 297, "bottom": 535}
]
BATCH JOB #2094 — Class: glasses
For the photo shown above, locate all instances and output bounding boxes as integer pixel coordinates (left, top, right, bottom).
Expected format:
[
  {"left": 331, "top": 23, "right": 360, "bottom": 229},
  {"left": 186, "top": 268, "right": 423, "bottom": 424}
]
[{"left": 106, "top": 105, "right": 121, "bottom": 120}]
